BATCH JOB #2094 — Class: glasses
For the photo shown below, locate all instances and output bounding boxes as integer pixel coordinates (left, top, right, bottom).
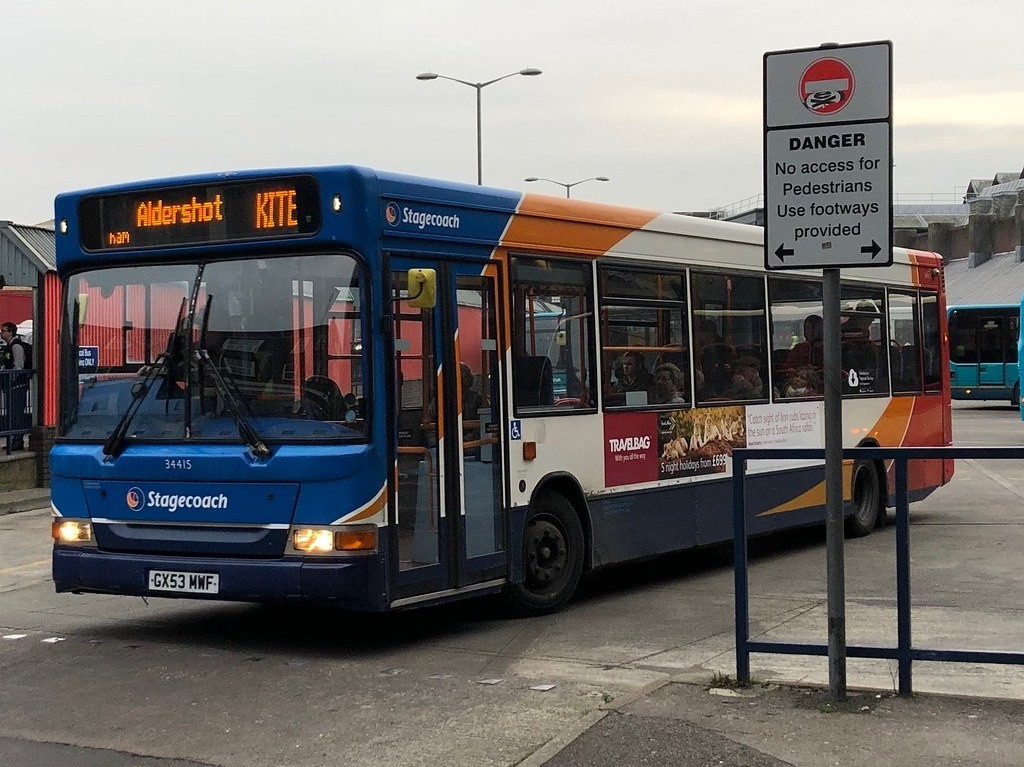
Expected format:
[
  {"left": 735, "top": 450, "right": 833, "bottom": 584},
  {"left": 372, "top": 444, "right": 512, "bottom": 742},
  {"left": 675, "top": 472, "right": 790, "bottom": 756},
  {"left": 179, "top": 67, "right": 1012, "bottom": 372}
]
[
  {"left": 1, "top": 329, "right": 8, "bottom": 333},
  {"left": 655, "top": 378, "right": 672, "bottom": 383}
]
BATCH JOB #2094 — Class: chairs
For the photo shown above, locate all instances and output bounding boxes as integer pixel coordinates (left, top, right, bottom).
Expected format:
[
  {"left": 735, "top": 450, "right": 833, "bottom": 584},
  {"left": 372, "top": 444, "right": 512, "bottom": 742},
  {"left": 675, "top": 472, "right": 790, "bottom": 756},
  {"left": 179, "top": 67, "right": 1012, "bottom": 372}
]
[{"left": 479, "top": 334, "right": 932, "bottom": 409}]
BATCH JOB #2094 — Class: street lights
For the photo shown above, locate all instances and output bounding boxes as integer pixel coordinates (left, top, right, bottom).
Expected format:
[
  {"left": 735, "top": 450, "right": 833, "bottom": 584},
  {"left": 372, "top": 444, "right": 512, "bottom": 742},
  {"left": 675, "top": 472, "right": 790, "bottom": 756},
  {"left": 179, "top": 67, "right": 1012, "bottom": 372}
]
[
  {"left": 524, "top": 176, "right": 610, "bottom": 198},
  {"left": 416, "top": 67, "right": 544, "bottom": 185}
]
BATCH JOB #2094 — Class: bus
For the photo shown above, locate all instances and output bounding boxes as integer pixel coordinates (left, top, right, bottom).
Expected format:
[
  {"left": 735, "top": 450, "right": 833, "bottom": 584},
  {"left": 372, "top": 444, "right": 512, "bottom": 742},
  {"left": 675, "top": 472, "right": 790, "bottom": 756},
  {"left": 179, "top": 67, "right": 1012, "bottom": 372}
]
[
  {"left": 45, "top": 158, "right": 955, "bottom": 616},
  {"left": 946, "top": 302, "right": 1020, "bottom": 409},
  {"left": 1018, "top": 295, "right": 1024, "bottom": 421}
]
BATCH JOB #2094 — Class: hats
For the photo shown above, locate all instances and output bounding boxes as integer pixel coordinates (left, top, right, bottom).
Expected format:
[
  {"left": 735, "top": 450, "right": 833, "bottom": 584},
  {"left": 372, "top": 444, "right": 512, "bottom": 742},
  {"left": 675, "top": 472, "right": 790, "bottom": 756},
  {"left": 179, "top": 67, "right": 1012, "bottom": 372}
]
[
  {"left": 731, "top": 356, "right": 760, "bottom": 366},
  {"left": 614, "top": 361, "right": 622, "bottom": 379}
]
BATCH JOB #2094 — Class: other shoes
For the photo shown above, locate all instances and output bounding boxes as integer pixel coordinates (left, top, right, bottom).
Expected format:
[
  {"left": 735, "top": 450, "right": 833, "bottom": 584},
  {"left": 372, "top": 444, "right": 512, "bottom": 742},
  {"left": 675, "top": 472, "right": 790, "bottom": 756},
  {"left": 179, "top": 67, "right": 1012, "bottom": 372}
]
[{"left": 2, "top": 439, "right": 25, "bottom": 451}]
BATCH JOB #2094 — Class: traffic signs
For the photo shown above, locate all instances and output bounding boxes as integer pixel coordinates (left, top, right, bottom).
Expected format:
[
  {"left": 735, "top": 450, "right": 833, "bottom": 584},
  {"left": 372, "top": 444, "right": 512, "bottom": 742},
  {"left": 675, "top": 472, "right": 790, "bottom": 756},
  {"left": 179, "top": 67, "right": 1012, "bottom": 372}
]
[{"left": 764, "top": 122, "right": 894, "bottom": 271}]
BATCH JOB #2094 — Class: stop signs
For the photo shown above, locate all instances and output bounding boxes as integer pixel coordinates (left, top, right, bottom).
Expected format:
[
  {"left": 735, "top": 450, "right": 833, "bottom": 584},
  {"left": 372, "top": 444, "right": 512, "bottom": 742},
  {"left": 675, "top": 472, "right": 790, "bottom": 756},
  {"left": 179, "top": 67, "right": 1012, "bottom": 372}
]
[{"left": 798, "top": 57, "right": 855, "bottom": 116}]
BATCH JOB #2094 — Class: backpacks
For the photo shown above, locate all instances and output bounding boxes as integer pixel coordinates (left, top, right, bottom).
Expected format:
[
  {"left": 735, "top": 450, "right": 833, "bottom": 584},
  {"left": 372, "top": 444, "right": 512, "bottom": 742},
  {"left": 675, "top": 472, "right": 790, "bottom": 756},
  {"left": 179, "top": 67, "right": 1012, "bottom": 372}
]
[{"left": 10, "top": 341, "right": 32, "bottom": 380}]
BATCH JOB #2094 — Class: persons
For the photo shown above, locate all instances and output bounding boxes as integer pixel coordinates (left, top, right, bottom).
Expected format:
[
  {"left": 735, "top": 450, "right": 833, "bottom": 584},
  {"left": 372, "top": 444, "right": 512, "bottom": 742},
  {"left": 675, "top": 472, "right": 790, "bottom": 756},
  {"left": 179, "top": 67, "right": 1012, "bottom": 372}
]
[
  {"left": 138, "top": 299, "right": 234, "bottom": 395},
  {"left": 0, "top": 321, "right": 29, "bottom": 451},
  {"left": 428, "top": 360, "right": 481, "bottom": 454},
  {"left": 611, "top": 300, "right": 879, "bottom": 400}
]
